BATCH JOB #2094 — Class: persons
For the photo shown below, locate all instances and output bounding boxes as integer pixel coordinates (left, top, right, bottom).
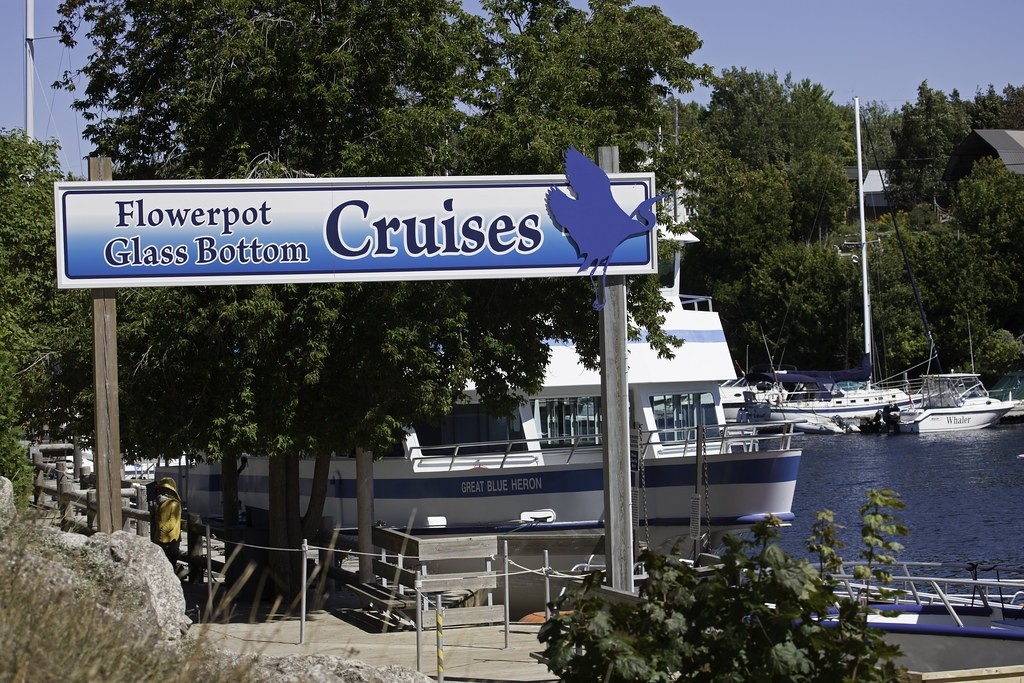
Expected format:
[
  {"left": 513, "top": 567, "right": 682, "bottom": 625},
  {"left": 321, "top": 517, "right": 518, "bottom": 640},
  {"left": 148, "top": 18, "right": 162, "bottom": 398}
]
[{"left": 152, "top": 475, "right": 184, "bottom": 573}]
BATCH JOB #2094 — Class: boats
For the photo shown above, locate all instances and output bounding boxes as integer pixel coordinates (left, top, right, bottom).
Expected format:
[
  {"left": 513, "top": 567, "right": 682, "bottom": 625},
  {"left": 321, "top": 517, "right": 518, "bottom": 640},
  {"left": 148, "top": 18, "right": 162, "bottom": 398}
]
[
  {"left": 644, "top": 374, "right": 820, "bottom": 420},
  {"left": 897, "top": 372, "right": 1024, "bottom": 441},
  {"left": 159, "top": 99, "right": 801, "bottom": 617}
]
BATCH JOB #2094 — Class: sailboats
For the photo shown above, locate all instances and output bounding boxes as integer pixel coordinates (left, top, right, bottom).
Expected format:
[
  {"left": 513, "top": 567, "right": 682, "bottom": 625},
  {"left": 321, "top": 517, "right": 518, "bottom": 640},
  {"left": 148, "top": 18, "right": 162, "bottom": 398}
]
[{"left": 741, "top": 99, "right": 930, "bottom": 436}]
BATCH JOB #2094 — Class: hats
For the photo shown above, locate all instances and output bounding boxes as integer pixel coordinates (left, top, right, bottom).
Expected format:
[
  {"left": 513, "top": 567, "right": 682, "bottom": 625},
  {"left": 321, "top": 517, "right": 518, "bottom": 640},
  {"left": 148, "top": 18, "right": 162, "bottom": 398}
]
[{"left": 154, "top": 476, "right": 180, "bottom": 500}]
